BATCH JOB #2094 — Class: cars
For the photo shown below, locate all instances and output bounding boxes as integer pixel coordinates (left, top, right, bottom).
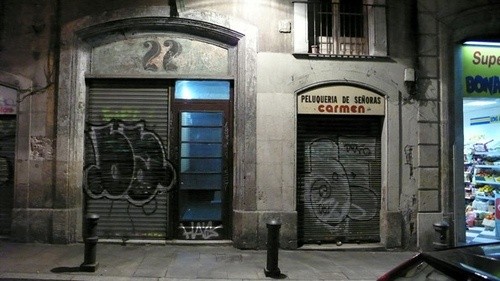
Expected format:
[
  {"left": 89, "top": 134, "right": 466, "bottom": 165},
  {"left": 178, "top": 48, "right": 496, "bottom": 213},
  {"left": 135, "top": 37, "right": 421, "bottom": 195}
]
[{"left": 374, "top": 239, "right": 500, "bottom": 281}]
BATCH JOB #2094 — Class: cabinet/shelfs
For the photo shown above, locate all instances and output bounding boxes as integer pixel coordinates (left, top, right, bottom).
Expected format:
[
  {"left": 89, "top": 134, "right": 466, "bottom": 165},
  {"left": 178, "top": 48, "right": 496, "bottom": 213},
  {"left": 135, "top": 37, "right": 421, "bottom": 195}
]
[{"left": 465, "top": 138, "right": 500, "bottom": 225}]
